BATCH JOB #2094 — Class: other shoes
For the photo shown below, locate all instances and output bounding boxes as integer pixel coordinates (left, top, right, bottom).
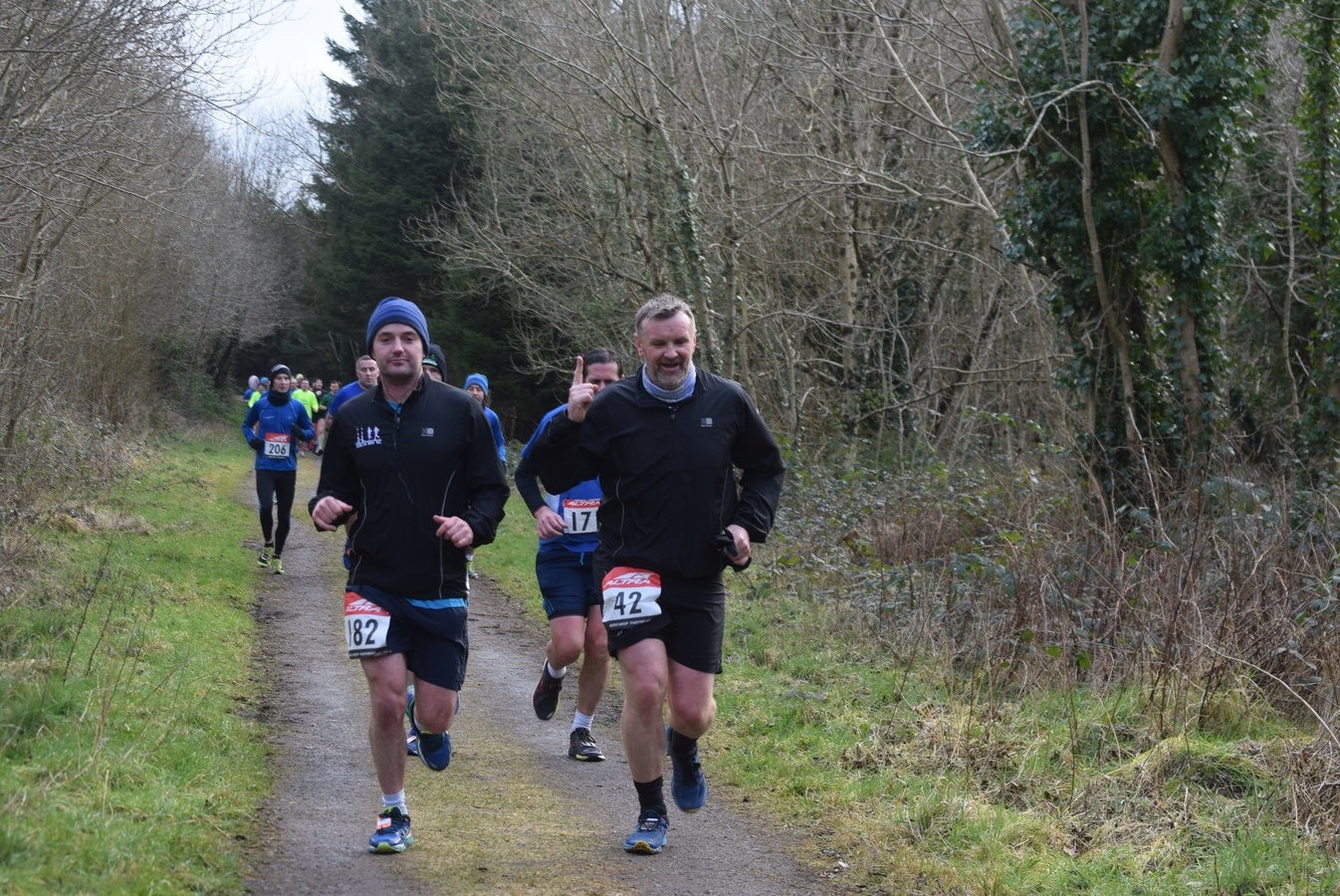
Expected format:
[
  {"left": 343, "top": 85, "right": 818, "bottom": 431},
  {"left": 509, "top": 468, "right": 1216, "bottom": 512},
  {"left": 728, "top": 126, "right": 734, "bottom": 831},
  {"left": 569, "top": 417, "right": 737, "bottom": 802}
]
[
  {"left": 316, "top": 448, "right": 323, "bottom": 456},
  {"left": 307, "top": 442, "right": 314, "bottom": 449}
]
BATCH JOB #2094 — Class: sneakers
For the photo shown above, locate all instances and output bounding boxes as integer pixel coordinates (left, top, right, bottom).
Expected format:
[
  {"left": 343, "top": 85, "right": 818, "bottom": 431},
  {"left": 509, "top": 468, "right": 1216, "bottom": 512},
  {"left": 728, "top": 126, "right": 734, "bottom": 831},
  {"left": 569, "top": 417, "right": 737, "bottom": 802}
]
[
  {"left": 272, "top": 558, "right": 283, "bottom": 574},
  {"left": 665, "top": 725, "right": 706, "bottom": 813},
  {"left": 569, "top": 728, "right": 605, "bottom": 761},
  {"left": 468, "top": 560, "right": 478, "bottom": 580},
  {"left": 532, "top": 659, "right": 567, "bottom": 721},
  {"left": 368, "top": 806, "right": 413, "bottom": 854},
  {"left": 406, "top": 727, "right": 419, "bottom": 756},
  {"left": 624, "top": 811, "right": 670, "bottom": 854},
  {"left": 344, "top": 540, "right": 353, "bottom": 569},
  {"left": 258, "top": 546, "right": 273, "bottom": 567},
  {"left": 405, "top": 685, "right": 451, "bottom": 772}
]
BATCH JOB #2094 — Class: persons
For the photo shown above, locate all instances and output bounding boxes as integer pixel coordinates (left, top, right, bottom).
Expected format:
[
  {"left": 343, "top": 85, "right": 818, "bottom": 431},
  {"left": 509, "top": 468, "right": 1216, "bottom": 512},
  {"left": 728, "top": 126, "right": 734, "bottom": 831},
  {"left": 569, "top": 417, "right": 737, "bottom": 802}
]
[
  {"left": 242, "top": 342, "right": 381, "bottom": 574},
  {"left": 422, "top": 342, "right": 448, "bottom": 384},
  {"left": 515, "top": 295, "right": 787, "bottom": 854},
  {"left": 306, "top": 294, "right": 510, "bottom": 853},
  {"left": 463, "top": 373, "right": 508, "bottom": 476}
]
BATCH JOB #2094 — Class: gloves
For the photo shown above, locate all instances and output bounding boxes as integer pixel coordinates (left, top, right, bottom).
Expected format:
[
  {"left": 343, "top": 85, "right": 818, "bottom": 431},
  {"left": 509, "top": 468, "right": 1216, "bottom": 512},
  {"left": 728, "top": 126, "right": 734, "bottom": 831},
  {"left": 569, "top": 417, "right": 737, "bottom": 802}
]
[
  {"left": 290, "top": 423, "right": 302, "bottom": 436},
  {"left": 251, "top": 439, "right": 265, "bottom": 451}
]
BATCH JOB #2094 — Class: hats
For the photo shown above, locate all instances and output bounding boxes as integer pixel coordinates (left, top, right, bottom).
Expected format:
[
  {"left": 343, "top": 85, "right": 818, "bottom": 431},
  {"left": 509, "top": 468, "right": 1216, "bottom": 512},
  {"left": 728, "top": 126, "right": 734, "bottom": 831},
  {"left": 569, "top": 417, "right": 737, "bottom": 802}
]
[
  {"left": 367, "top": 297, "right": 430, "bottom": 356},
  {"left": 295, "top": 373, "right": 304, "bottom": 380},
  {"left": 462, "top": 373, "right": 489, "bottom": 396},
  {"left": 422, "top": 344, "right": 447, "bottom": 382},
  {"left": 271, "top": 365, "right": 292, "bottom": 383}
]
